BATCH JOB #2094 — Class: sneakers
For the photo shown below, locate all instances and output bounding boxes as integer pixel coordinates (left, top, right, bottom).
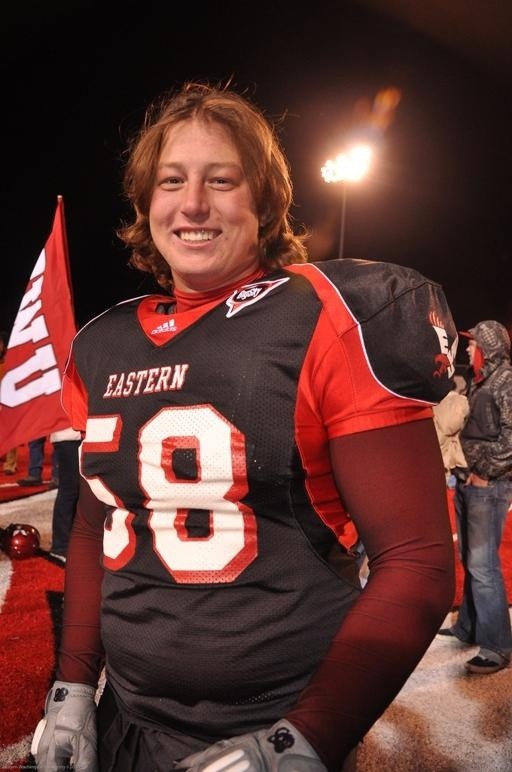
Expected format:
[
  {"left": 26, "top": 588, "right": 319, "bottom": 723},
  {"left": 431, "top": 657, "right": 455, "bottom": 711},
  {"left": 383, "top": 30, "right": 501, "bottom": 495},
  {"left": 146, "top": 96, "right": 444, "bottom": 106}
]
[
  {"left": 464, "top": 654, "right": 506, "bottom": 674},
  {"left": 16, "top": 478, "right": 58, "bottom": 489},
  {"left": 435, "top": 628, "right": 463, "bottom": 642}
]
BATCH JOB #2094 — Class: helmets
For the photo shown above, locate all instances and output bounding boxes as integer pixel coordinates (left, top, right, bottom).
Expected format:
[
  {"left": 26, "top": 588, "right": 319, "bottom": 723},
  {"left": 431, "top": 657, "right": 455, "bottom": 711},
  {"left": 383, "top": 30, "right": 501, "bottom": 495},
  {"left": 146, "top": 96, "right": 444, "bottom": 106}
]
[{"left": 4, "top": 524, "right": 41, "bottom": 560}]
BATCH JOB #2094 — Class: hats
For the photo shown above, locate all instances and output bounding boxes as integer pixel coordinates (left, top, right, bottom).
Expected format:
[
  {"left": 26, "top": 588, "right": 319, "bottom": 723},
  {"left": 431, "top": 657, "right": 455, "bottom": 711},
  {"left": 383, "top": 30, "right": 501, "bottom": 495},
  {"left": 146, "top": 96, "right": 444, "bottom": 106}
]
[{"left": 458, "top": 330, "right": 473, "bottom": 339}]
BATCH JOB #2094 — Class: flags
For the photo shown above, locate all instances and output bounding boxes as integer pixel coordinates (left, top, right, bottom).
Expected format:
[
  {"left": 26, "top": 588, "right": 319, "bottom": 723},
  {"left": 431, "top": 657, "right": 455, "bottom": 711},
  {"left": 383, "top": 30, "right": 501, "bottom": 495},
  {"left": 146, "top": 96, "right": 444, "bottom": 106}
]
[{"left": 0, "top": 199, "right": 76, "bottom": 455}]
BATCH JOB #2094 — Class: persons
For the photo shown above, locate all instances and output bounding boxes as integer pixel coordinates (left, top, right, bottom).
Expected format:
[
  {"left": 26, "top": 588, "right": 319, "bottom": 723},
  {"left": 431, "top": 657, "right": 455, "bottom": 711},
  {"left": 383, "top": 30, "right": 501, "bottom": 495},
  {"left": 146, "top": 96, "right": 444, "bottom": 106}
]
[
  {"left": 1, "top": 321, "right": 511, "bottom": 674},
  {"left": 30, "top": 83, "right": 459, "bottom": 772}
]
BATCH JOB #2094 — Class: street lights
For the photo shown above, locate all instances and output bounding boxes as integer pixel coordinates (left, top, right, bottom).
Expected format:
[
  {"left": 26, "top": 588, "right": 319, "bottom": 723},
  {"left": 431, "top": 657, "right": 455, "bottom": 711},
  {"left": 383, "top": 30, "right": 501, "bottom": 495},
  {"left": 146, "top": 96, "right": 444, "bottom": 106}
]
[{"left": 321, "top": 143, "right": 373, "bottom": 259}]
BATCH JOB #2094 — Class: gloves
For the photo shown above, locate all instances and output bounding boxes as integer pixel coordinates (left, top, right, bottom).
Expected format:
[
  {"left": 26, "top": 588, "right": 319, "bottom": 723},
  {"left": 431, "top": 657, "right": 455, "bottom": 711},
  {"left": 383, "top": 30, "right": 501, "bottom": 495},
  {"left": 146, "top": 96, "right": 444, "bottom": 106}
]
[
  {"left": 175, "top": 718, "right": 330, "bottom": 772},
  {"left": 30, "top": 681, "right": 98, "bottom": 772}
]
